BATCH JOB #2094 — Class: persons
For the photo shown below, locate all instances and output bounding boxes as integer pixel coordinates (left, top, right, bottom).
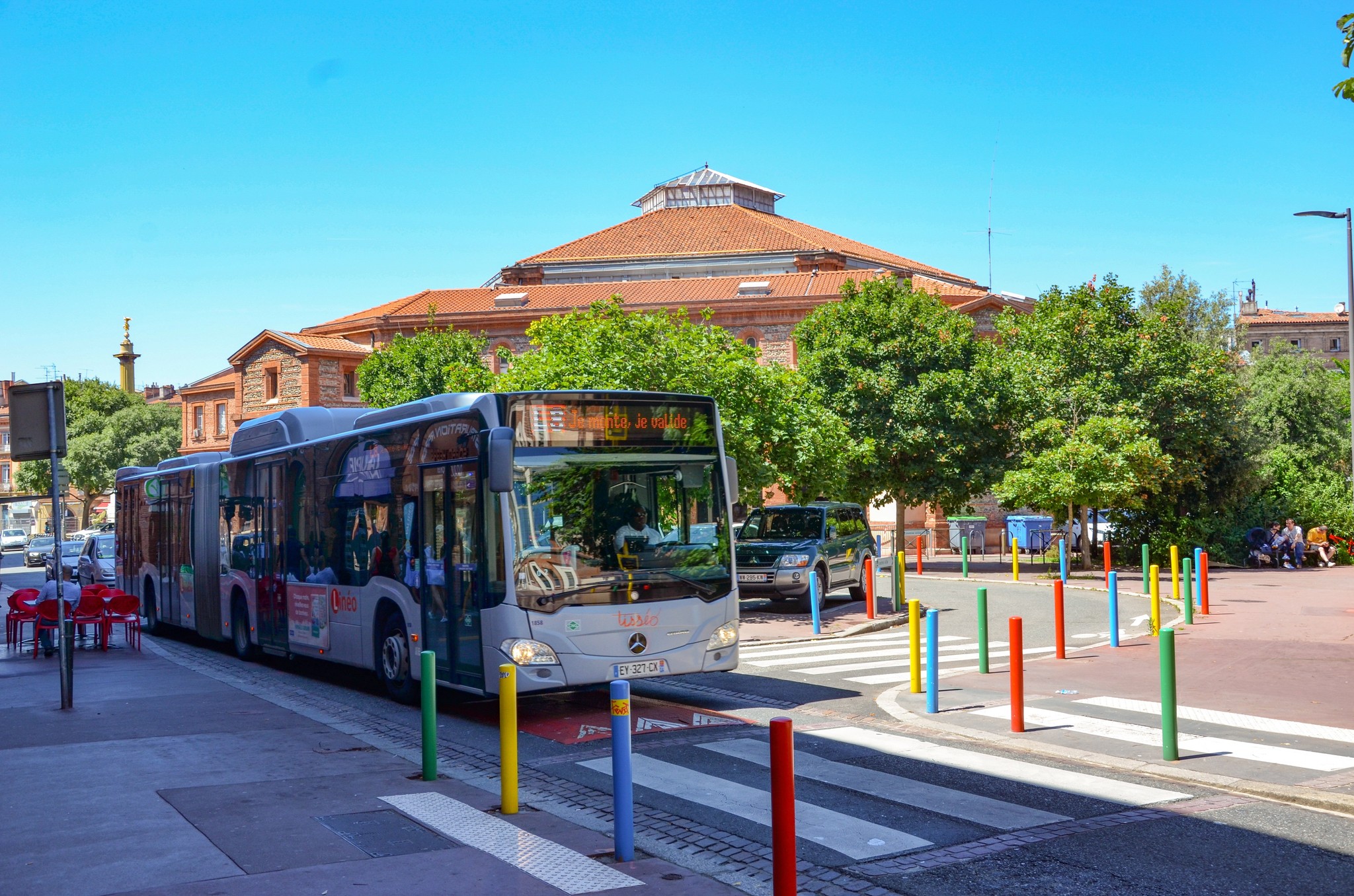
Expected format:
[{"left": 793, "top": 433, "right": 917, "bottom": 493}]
[
  {"left": 222, "top": 530, "right": 477, "bottom": 622},
  {"left": 1305, "top": 524, "right": 1336, "bottom": 568},
  {"left": 35, "top": 564, "right": 81, "bottom": 659},
  {"left": 614, "top": 504, "right": 663, "bottom": 570},
  {"left": 1272, "top": 533, "right": 1289, "bottom": 544},
  {"left": 44, "top": 522, "right": 50, "bottom": 537},
  {"left": 1265, "top": 522, "right": 1295, "bottom": 569},
  {"left": 1281, "top": 518, "right": 1307, "bottom": 569}
]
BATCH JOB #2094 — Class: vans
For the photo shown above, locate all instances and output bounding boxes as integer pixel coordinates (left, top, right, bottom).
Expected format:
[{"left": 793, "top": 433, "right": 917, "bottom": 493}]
[
  {"left": 1097, "top": 508, "right": 1151, "bottom": 540},
  {"left": 1058, "top": 508, "right": 1124, "bottom": 552}
]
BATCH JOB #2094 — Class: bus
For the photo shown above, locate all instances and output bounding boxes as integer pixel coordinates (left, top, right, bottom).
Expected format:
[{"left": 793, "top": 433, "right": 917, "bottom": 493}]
[{"left": 114, "top": 391, "right": 741, "bottom": 707}]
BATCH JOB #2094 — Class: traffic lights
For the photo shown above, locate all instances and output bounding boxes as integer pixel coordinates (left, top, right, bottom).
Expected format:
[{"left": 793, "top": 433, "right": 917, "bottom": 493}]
[{"left": 65, "top": 503, "right": 67, "bottom": 511}]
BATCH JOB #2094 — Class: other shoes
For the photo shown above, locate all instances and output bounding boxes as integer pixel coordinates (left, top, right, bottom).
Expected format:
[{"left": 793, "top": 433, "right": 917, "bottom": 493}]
[
  {"left": 428, "top": 611, "right": 436, "bottom": 619},
  {"left": 441, "top": 617, "right": 447, "bottom": 623},
  {"left": 44, "top": 648, "right": 54, "bottom": 656}
]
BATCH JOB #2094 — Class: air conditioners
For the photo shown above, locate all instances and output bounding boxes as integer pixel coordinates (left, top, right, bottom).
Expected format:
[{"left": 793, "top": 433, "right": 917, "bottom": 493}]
[
  {"left": 4, "top": 484, "right": 12, "bottom": 492},
  {"left": 193, "top": 429, "right": 200, "bottom": 437}
]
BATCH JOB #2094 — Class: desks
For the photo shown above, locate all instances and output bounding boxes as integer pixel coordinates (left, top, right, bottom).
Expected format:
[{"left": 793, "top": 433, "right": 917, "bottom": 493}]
[{"left": 24, "top": 597, "right": 124, "bottom": 652}]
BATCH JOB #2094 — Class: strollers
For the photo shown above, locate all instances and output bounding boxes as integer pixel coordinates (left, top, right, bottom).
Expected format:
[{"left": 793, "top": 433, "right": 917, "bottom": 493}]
[{"left": 1243, "top": 527, "right": 1280, "bottom": 569}]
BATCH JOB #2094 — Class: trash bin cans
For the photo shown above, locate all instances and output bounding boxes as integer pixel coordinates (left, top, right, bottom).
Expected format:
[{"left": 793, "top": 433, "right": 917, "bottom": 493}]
[
  {"left": 1003, "top": 515, "right": 1054, "bottom": 549},
  {"left": 946, "top": 515, "right": 989, "bottom": 549}
]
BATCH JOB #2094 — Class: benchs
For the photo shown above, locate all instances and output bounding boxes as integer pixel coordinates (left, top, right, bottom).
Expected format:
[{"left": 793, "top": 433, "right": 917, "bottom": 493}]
[{"left": 1275, "top": 539, "right": 1332, "bottom": 567}]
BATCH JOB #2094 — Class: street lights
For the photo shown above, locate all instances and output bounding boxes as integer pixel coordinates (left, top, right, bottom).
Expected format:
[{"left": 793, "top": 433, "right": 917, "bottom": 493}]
[{"left": 1293, "top": 207, "right": 1354, "bottom": 477}]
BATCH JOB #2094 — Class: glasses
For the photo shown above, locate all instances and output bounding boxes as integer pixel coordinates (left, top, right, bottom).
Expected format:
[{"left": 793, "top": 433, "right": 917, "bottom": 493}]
[{"left": 635, "top": 512, "right": 648, "bottom": 517}]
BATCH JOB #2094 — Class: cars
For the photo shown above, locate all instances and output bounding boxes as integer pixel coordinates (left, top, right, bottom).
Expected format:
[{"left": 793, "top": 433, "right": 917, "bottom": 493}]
[
  {"left": 0, "top": 528, "right": 31, "bottom": 552},
  {"left": 22, "top": 536, "right": 63, "bottom": 568},
  {"left": 662, "top": 522, "right": 764, "bottom": 547},
  {"left": 45, "top": 541, "right": 86, "bottom": 585},
  {"left": 231, "top": 533, "right": 262, "bottom": 580}
]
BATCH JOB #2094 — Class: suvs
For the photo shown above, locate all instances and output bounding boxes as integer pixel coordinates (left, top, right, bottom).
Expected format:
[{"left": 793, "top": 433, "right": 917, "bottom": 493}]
[
  {"left": 735, "top": 500, "right": 876, "bottom": 614},
  {"left": 76, "top": 533, "right": 115, "bottom": 590},
  {"left": 73, "top": 522, "right": 115, "bottom": 542}
]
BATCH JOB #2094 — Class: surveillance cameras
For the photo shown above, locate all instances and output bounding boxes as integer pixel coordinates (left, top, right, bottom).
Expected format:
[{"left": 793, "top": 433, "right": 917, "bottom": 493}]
[{"left": 672, "top": 469, "right": 682, "bottom": 481}]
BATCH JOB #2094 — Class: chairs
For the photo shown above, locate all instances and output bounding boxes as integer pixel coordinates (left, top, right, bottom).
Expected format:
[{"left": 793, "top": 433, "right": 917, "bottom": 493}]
[{"left": 6, "top": 584, "right": 142, "bottom": 660}]
